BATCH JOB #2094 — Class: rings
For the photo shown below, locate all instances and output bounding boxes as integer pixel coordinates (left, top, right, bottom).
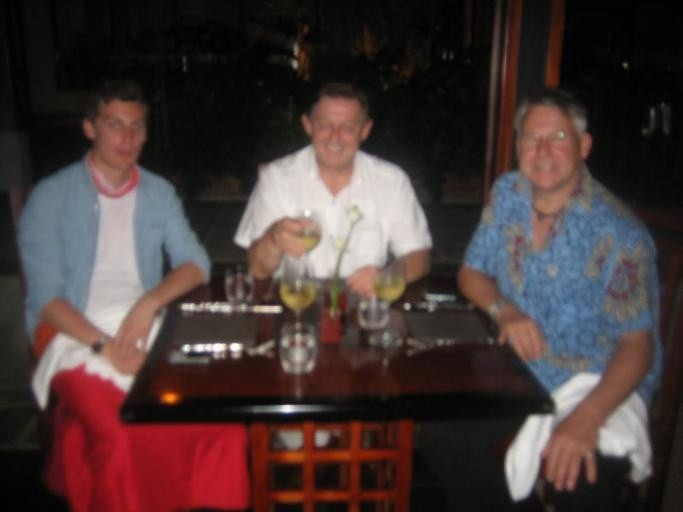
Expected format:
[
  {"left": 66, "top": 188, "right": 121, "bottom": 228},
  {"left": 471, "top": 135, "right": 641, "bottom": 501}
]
[{"left": 583, "top": 451, "right": 596, "bottom": 461}]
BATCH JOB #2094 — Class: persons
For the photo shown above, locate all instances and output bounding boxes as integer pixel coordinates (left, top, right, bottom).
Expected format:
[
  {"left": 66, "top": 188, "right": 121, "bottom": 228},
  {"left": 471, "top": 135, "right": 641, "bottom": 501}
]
[
  {"left": 416, "top": 89, "right": 670, "bottom": 512},
  {"left": 232, "top": 80, "right": 436, "bottom": 298},
  {"left": 17, "top": 79, "right": 255, "bottom": 512}
]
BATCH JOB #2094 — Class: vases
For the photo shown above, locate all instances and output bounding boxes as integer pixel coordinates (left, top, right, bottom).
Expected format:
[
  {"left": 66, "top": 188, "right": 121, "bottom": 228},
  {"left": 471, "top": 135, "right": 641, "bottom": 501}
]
[{"left": 317, "top": 306, "right": 343, "bottom": 346}]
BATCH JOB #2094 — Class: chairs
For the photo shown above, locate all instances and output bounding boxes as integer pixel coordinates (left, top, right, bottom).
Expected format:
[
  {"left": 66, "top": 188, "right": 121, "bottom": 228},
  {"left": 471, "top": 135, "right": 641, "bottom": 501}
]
[
  {"left": 540, "top": 243, "right": 681, "bottom": 511},
  {"left": 6, "top": 183, "right": 60, "bottom": 451},
  {"left": 245, "top": 418, "right": 416, "bottom": 510}
]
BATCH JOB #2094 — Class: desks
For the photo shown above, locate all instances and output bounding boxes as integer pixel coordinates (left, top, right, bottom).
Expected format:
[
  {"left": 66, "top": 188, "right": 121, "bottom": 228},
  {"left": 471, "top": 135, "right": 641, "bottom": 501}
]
[{"left": 117, "top": 280, "right": 559, "bottom": 511}]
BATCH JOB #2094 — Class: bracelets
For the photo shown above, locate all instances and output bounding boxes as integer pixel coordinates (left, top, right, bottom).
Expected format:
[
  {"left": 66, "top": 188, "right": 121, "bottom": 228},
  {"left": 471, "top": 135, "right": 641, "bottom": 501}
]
[
  {"left": 486, "top": 298, "right": 512, "bottom": 321},
  {"left": 92, "top": 333, "right": 113, "bottom": 351}
]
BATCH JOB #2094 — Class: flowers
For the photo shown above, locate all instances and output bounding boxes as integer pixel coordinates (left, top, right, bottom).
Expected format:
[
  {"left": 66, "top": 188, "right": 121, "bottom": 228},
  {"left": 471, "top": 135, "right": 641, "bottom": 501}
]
[{"left": 328, "top": 203, "right": 365, "bottom": 314}]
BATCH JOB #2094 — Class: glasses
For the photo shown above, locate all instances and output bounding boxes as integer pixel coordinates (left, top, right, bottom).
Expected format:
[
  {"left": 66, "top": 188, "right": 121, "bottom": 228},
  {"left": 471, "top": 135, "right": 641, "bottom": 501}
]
[{"left": 517, "top": 128, "right": 579, "bottom": 147}]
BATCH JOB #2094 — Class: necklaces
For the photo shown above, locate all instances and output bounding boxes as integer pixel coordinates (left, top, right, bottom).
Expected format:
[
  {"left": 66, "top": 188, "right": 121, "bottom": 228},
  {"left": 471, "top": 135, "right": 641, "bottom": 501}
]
[
  {"left": 531, "top": 205, "right": 559, "bottom": 222},
  {"left": 94, "top": 162, "right": 126, "bottom": 187}
]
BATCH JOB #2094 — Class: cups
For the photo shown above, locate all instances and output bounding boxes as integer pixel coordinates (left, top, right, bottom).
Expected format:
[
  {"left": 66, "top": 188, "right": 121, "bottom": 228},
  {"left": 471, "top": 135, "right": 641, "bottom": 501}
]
[{"left": 279, "top": 321, "right": 318, "bottom": 379}]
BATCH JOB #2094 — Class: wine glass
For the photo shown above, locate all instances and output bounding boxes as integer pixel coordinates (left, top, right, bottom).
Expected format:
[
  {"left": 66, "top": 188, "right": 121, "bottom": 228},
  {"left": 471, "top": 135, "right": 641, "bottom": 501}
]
[{"left": 224, "top": 205, "right": 405, "bottom": 347}]
